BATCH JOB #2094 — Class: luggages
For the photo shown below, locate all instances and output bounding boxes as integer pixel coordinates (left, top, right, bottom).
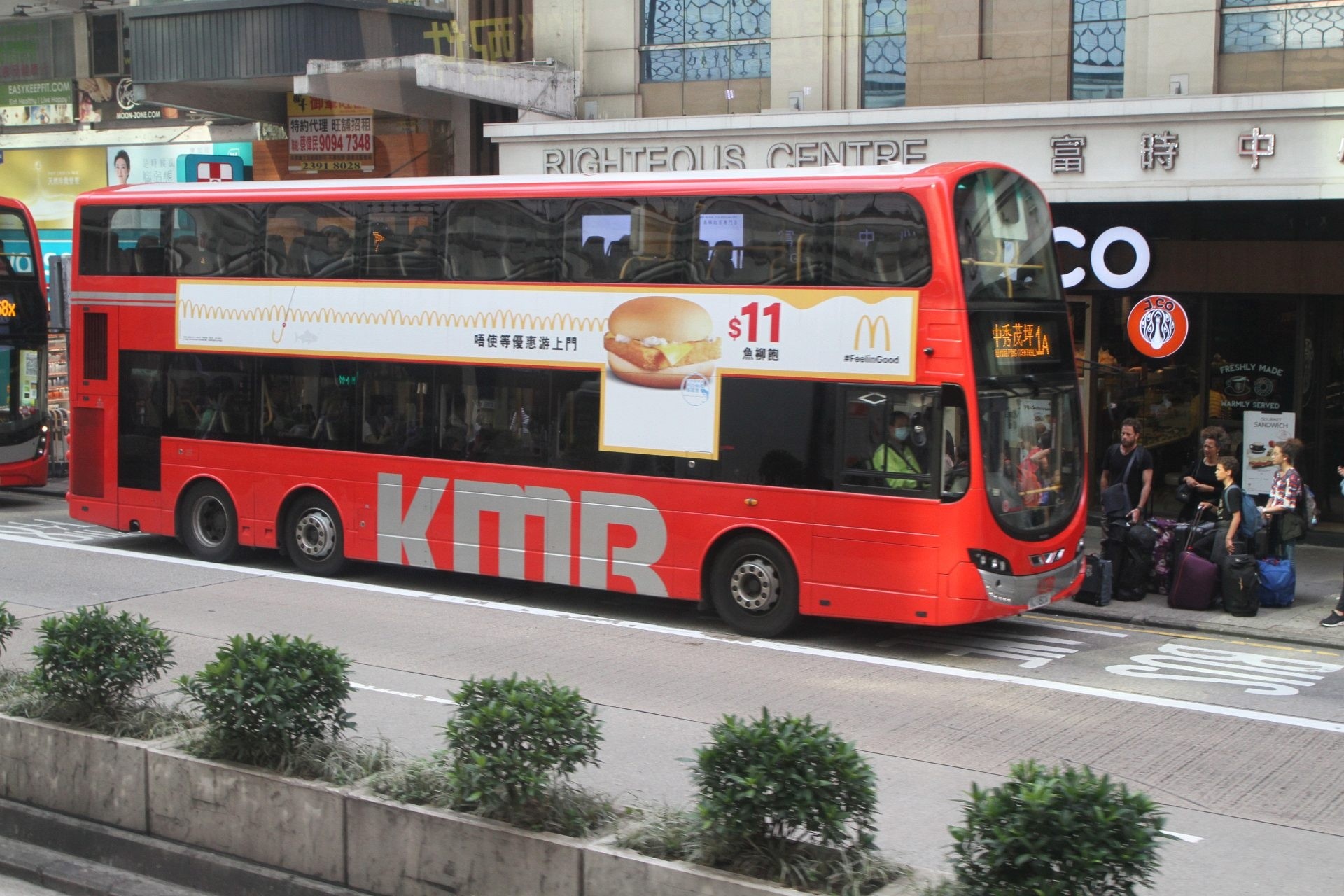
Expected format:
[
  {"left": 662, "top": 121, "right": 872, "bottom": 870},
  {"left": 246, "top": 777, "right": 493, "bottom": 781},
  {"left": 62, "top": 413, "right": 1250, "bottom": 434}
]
[
  {"left": 1222, "top": 542, "right": 1264, "bottom": 618},
  {"left": 1168, "top": 507, "right": 1219, "bottom": 612},
  {"left": 1105, "top": 483, "right": 1216, "bottom": 602}
]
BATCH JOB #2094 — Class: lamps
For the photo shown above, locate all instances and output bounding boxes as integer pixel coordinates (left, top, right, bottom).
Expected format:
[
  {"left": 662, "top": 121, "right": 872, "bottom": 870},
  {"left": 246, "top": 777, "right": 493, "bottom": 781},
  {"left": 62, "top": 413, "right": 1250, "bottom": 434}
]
[{"left": 11, "top": 0, "right": 114, "bottom": 17}]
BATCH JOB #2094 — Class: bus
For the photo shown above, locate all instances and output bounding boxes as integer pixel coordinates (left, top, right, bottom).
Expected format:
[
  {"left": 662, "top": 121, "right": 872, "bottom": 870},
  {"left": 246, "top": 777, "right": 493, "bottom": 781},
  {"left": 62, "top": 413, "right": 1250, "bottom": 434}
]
[
  {"left": 0, "top": 196, "right": 52, "bottom": 489},
  {"left": 62, "top": 158, "right": 1140, "bottom": 640}
]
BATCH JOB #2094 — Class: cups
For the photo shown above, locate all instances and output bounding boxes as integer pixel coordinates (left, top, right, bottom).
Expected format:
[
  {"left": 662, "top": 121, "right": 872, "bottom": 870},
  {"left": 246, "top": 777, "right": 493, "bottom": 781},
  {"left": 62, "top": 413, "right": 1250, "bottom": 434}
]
[
  {"left": 1002, "top": 500, "right": 1023, "bottom": 512},
  {"left": 1249, "top": 443, "right": 1264, "bottom": 450}
]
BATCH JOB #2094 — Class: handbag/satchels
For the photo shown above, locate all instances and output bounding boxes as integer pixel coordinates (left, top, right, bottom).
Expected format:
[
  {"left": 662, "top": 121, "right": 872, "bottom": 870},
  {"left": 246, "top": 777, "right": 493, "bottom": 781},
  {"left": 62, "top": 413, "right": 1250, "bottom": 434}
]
[
  {"left": 1225, "top": 486, "right": 1263, "bottom": 539},
  {"left": 1073, "top": 553, "right": 1113, "bottom": 607},
  {"left": 1174, "top": 484, "right": 1193, "bottom": 504},
  {"left": 1257, "top": 558, "right": 1297, "bottom": 608},
  {"left": 1102, "top": 485, "right": 1132, "bottom": 517}
]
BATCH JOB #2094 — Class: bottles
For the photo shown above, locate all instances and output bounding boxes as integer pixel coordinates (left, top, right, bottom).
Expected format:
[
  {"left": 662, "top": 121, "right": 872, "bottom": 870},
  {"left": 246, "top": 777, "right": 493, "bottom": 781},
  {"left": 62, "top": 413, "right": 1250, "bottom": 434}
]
[
  {"left": 1311, "top": 515, "right": 1317, "bottom": 524},
  {"left": 47, "top": 376, "right": 69, "bottom": 400},
  {"left": 48, "top": 352, "right": 67, "bottom": 373}
]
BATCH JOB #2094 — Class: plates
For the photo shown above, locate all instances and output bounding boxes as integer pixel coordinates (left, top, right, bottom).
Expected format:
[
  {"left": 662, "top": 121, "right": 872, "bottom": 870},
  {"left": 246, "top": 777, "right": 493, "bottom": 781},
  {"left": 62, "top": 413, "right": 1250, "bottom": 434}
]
[{"left": 1250, "top": 448, "right": 1266, "bottom": 453}]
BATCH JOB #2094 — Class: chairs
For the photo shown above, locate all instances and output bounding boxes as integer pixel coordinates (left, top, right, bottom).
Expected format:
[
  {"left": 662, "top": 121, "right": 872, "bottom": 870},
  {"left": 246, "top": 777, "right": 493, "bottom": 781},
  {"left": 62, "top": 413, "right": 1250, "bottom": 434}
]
[
  {"left": 128, "top": 375, "right": 522, "bottom": 464},
  {"left": 101, "top": 231, "right": 902, "bottom": 284}
]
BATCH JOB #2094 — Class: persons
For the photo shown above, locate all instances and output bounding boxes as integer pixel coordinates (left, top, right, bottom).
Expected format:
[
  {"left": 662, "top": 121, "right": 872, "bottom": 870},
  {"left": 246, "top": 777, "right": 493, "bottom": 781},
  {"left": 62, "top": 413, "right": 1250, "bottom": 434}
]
[
  {"left": 945, "top": 425, "right": 1067, "bottom": 525},
  {"left": 308, "top": 224, "right": 439, "bottom": 279},
  {"left": 109, "top": 150, "right": 140, "bottom": 228},
  {"left": 127, "top": 376, "right": 601, "bottom": 471},
  {"left": 1101, "top": 385, "right": 1344, "bottom": 627},
  {"left": 873, "top": 411, "right": 932, "bottom": 491}
]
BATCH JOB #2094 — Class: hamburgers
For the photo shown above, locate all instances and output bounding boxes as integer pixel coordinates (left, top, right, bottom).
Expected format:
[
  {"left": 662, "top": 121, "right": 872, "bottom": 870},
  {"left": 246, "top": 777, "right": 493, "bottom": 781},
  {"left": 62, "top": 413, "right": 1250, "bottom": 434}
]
[{"left": 603, "top": 296, "right": 721, "bottom": 390}]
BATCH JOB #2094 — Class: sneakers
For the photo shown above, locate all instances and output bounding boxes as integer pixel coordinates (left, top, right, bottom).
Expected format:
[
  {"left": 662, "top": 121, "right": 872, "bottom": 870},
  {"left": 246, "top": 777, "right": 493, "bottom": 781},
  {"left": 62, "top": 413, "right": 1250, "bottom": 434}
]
[{"left": 1320, "top": 609, "right": 1344, "bottom": 627}]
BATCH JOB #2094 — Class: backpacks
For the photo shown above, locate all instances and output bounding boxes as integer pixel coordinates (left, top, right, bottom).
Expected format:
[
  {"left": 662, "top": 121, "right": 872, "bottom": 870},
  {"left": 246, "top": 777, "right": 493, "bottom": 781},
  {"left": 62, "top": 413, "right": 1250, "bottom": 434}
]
[{"left": 1301, "top": 483, "right": 1319, "bottom": 528}]
